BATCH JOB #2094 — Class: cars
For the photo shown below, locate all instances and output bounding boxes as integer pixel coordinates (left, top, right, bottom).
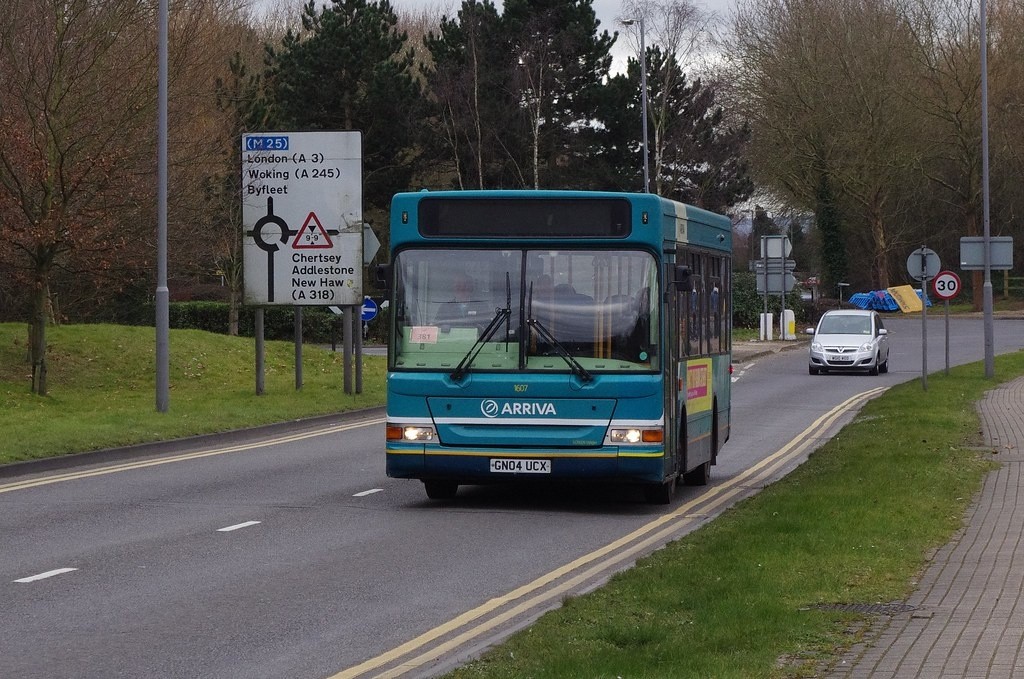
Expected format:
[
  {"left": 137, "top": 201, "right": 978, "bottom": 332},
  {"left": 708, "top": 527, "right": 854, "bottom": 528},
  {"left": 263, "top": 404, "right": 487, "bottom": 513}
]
[
  {"left": 806, "top": 310, "right": 891, "bottom": 375},
  {"left": 800, "top": 277, "right": 820, "bottom": 290}
]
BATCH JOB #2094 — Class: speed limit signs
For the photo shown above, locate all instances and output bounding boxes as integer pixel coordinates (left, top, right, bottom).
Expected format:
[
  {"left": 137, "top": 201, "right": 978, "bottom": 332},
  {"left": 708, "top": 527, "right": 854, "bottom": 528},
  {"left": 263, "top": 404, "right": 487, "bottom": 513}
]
[{"left": 932, "top": 272, "right": 961, "bottom": 298}]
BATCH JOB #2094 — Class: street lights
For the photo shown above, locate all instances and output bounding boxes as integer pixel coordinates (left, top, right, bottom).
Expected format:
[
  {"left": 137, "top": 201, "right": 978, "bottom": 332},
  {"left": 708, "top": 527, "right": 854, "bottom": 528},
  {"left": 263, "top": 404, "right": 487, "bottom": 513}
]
[{"left": 623, "top": 18, "right": 650, "bottom": 191}]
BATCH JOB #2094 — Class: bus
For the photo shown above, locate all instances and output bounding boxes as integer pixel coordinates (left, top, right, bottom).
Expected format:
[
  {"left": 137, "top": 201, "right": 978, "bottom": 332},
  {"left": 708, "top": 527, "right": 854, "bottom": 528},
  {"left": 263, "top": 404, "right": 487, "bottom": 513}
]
[{"left": 375, "top": 189, "right": 733, "bottom": 501}]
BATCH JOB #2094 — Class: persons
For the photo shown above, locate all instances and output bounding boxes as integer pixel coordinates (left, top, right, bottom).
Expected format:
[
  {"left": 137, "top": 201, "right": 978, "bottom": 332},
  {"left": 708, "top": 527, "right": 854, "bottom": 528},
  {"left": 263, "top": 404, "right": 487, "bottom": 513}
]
[
  {"left": 433, "top": 275, "right": 492, "bottom": 335},
  {"left": 621, "top": 287, "right": 650, "bottom": 365},
  {"left": 681, "top": 314, "right": 689, "bottom": 357},
  {"left": 691, "top": 285, "right": 720, "bottom": 342}
]
